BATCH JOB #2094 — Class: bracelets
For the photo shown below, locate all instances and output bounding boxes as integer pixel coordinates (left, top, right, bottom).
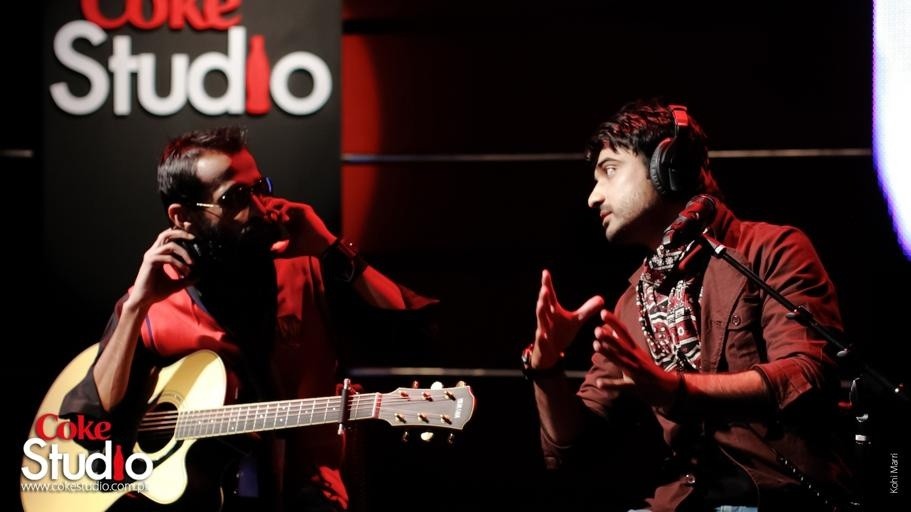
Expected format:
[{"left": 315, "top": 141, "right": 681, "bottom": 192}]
[
  {"left": 314, "top": 234, "right": 368, "bottom": 288},
  {"left": 520, "top": 344, "right": 569, "bottom": 380},
  {"left": 650, "top": 366, "right": 690, "bottom": 422}
]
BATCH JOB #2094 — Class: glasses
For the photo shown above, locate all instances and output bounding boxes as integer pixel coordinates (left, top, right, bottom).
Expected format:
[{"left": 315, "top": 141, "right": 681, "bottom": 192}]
[{"left": 197, "top": 176, "right": 272, "bottom": 210}]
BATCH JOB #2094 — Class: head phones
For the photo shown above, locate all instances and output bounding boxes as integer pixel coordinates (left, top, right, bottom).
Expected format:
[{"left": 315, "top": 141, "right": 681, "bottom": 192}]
[{"left": 649, "top": 102, "right": 705, "bottom": 199}]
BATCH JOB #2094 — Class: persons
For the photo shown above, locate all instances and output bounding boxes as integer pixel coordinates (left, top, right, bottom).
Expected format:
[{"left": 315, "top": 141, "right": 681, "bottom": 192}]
[
  {"left": 526, "top": 100, "right": 842, "bottom": 511},
  {"left": 62, "top": 127, "right": 445, "bottom": 511}
]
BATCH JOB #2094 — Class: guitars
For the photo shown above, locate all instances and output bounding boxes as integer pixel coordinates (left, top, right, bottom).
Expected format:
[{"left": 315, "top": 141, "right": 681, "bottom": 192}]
[{"left": 19, "top": 342, "right": 476, "bottom": 512}]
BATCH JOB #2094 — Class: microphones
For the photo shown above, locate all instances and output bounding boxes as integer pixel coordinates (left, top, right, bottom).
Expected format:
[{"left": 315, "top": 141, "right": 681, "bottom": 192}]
[{"left": 661, "top": 195, "right": 717, "bottom": 252}]
